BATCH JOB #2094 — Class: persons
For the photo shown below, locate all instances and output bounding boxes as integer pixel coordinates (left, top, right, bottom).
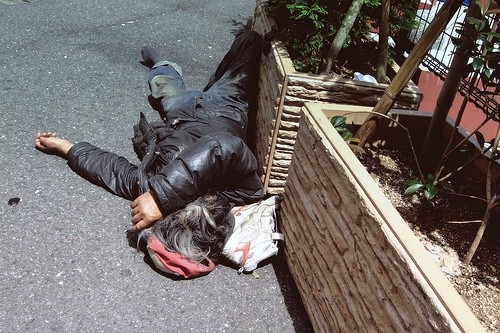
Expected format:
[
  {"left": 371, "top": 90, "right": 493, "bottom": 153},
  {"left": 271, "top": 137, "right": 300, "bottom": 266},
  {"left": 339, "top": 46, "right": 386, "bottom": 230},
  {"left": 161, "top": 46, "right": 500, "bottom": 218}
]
[{"left": 33, "top": 19, "right": 267, "bottom": 277}]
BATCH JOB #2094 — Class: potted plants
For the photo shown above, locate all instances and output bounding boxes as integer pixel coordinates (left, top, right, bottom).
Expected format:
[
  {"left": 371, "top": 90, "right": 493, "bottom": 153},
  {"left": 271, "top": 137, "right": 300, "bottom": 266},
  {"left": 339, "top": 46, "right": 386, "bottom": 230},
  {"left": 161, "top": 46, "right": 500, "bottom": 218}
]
[
  {"left": 277, "top": 0, "right": 500, "bottom": 333},
  {"left": 251, "top": 0, "right": 422, "bottom": 193}
]
